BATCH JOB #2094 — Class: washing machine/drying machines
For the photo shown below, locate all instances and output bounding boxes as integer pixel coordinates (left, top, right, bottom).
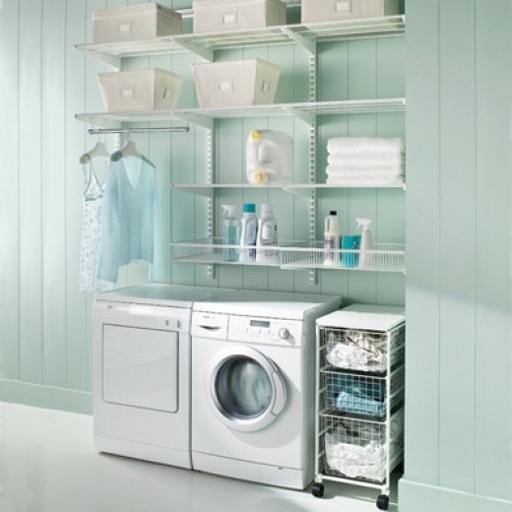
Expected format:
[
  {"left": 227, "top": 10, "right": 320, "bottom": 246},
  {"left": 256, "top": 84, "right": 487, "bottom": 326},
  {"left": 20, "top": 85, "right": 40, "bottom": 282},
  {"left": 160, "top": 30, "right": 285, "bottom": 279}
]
[
  {"left": 190, "top": 288, "right": 342, "bottom": 492},
  {"left": 90, "top": 281, "right": 240, "bottom": 473}
]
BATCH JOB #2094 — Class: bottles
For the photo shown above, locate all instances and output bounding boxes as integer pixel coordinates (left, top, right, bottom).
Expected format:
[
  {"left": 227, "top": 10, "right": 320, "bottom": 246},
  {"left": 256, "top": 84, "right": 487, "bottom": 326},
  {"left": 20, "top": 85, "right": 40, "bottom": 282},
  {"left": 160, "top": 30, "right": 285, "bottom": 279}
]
[
  {"left": 258, "top": 207, "right": 280, "bottom": 263},
  {"left": 324, "top": 209, "right": 340, "bottom": 266}
]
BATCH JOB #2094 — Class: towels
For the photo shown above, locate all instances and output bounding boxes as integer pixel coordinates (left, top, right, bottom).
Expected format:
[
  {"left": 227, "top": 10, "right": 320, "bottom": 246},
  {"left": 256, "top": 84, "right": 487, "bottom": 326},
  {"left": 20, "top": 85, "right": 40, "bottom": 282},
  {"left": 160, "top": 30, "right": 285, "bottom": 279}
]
[{"left": 323, "top": 135, "right": 405, "bottom": 189}]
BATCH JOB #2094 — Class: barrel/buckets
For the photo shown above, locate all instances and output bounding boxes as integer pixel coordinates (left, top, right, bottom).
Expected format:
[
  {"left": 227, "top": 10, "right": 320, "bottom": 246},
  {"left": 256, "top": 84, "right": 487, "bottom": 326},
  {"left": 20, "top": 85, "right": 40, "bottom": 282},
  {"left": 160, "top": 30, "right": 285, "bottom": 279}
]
[{"left": 245, "top": 128, "right": 295, "bottom": 187}]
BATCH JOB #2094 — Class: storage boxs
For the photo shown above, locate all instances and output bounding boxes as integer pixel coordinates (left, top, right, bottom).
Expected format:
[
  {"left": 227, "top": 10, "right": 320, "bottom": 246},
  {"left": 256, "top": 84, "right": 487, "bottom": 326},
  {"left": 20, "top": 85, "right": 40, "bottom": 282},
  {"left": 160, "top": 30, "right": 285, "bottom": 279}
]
[
  {"left": 189, "top": 0, "right": 287, "bottom": 34},
  {"left": 190, "top": 58, "right": 284, "bottom": 109},
  {"left": 298, "top": 2, "right": 399, "bottom": 21},
  {"left": 87, "top": 3, "right": 183, "bottom": 43},
  {"left": 96, "top": 69, "right": 179, "bottom": 111}
]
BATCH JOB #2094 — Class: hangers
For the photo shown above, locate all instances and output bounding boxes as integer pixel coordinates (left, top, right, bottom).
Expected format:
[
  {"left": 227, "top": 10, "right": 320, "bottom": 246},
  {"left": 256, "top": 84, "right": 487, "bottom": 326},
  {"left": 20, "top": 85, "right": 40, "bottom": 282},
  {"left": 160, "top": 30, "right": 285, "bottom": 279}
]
[
  {"left": 78, "top": 130, "right": 109, "bottom": 167},
  {"left": 81, "top": 152, "right": 106, "bottom": 203},
  {"left": 111, "top": 126, "right": 160, "bottom": 173}
]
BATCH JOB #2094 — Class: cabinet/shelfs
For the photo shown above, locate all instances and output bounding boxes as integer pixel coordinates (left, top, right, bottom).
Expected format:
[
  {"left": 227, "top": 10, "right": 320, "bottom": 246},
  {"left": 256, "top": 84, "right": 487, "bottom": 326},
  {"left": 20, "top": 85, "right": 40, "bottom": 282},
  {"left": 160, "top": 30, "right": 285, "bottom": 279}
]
[
  {"left": 77, "top": 14, "right": 405, "bottom": 272},
  {"left": 314, "top": 302, "right": 407, "bottom": 492}
]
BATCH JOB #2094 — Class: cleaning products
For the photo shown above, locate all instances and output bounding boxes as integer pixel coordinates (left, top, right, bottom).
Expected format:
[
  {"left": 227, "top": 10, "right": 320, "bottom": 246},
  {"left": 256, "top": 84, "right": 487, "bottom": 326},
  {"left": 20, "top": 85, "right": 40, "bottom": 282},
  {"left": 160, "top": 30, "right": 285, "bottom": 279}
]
[
  {"left": 354, "top": 217, "right": 377, "bottom": 269},
  {"left": 322, "top": 210, "right": 341, "bottom": 267},
  {"left": 255, "top": 204, "right": 281, "bottom": 265},
  {"left": 221, "top": 202, "right": 240, "bottom": 261}
]
